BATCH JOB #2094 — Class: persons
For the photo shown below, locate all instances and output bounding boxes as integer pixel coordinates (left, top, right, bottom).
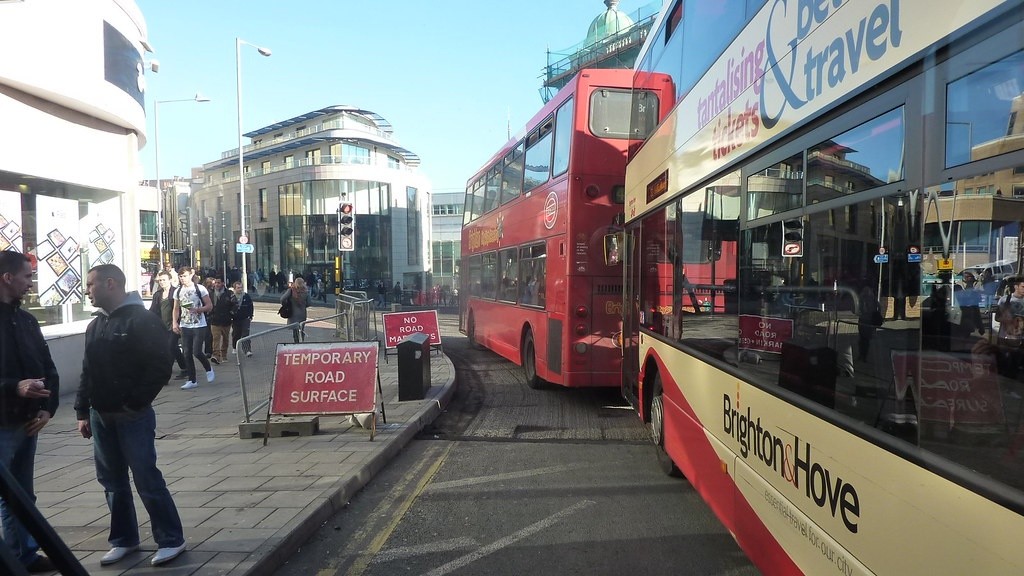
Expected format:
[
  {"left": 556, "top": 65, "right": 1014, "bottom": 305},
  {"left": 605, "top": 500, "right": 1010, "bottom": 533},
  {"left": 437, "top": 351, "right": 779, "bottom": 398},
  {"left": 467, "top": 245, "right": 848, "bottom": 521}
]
[
  {"left": 394, "top": 281, "right": 401, "bottom": 303},
  {"left": 0, "top": 250, "right": 60, "bottom": 571},
  {"left": 149, "top": 262, "right": 254, "bottom": 389},
  {"left": 248, "top": 267, "right": 327, "bottom": 300},
  {"left": 74, "top": 264, "right": 187, "bottom": 564},
  {"left": 856, "top": 272, "right": 1024, "bottom": 378},
  {"left": 376, "top": 281, "right": 385, "bottom": 309},
  {"left": 280, "top": 278, "right": 311, "bottom": 343}
]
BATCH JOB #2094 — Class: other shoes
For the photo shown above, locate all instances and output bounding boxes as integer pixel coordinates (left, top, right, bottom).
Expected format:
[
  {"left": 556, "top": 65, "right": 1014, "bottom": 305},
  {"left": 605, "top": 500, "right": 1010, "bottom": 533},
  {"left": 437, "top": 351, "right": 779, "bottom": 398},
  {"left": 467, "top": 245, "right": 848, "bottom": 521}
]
[
  {"left": 211, "top": 357, "right": 220, "bottom": 365},
  {"left": 222, "top": 359, "right": 228, "bottom": 363},
  {"left": 856, "top": 357, "right": 867, "bottom": 363},
  {"left": 376, "top": 306, "right": 386, "bottom": 309},
  {"left": 176, "top": 370, "right": 188, "bottom": 379},
  {"left": 30, "top": 555, "right": 58, "bottom": 572},
  {"left": 246, "top": 350, "right": 253, "bottom": 358},
  {"left": 232, "top": 349, "right": 237, "bottom": 354}
]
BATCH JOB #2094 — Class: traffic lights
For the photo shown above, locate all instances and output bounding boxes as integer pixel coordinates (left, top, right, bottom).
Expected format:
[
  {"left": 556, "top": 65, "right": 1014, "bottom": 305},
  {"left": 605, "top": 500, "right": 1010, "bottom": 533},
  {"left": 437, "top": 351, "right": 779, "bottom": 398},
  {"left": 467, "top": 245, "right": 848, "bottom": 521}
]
[
  {"left": 179, "top": 207, "right": 191, "bottom": 234},
  {"left": 337, "top": 201, "right": 354, "bottom": 252}
]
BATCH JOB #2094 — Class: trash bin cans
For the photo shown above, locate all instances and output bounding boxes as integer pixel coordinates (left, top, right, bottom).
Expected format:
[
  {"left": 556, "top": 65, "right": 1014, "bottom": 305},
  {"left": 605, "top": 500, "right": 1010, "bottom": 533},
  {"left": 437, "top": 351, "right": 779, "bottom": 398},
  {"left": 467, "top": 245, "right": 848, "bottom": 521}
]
[{"left": 396, "top": 332, "right": 432, "bottom": 401}]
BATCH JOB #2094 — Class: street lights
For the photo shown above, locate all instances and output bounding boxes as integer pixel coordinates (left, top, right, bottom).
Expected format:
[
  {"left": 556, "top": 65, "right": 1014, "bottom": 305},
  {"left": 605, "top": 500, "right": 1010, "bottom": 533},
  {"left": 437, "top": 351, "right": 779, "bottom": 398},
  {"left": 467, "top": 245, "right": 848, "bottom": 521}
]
[
  {"left": 236, "top": 38, "right": 271, "bottom": 290},
  {"left": 153, "top": 94, "right": 212, "bottom": 268}
]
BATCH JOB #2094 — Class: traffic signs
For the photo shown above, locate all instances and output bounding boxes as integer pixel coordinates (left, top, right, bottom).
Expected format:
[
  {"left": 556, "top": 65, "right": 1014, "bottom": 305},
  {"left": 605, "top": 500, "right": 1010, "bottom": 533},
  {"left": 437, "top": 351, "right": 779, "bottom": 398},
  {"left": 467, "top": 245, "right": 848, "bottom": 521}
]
[{"left": 236, "top": 243, "right": 254, "bottom": 253}]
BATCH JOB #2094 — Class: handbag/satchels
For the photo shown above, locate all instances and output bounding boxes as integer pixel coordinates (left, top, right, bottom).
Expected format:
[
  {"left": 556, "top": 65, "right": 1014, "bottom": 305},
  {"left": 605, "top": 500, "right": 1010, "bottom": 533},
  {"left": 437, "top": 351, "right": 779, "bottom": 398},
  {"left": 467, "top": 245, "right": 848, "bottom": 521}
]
[
  {"left": 871, "top": 311, "right": 883, "bottom": 326},
  {"left": 277, "top": 289, "right": 293, "bottom": 318}
]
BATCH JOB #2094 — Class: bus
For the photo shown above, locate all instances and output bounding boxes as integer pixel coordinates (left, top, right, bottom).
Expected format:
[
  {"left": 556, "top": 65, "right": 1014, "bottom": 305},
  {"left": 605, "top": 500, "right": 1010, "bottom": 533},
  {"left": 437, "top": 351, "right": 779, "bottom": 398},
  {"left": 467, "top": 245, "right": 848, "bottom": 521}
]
[
  {"left": 454, "top": 68, "right": 737, "bottom": 389},
  {"left": 623, "top": 0, "right": 1024, "bottom": 576},
  {"left": 629, "top": 73, "right": 676, "bottom": 339}
]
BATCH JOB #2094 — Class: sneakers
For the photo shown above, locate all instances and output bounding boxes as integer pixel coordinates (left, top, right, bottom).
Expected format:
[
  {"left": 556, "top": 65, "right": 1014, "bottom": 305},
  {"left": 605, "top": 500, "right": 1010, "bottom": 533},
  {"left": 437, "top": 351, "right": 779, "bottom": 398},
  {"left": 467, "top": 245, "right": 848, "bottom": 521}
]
[
  {"left": 100, "top": 544, "right": 139, "bottom": 565},
  {"left": 151, "top": 541, "right": 187, "bottom": 566},
  {"left": 206, "top": 366, "right": 216, "bottom": 383},
  {"left": 180, "top": 381, "right": 199, "bottom": 390}
]
